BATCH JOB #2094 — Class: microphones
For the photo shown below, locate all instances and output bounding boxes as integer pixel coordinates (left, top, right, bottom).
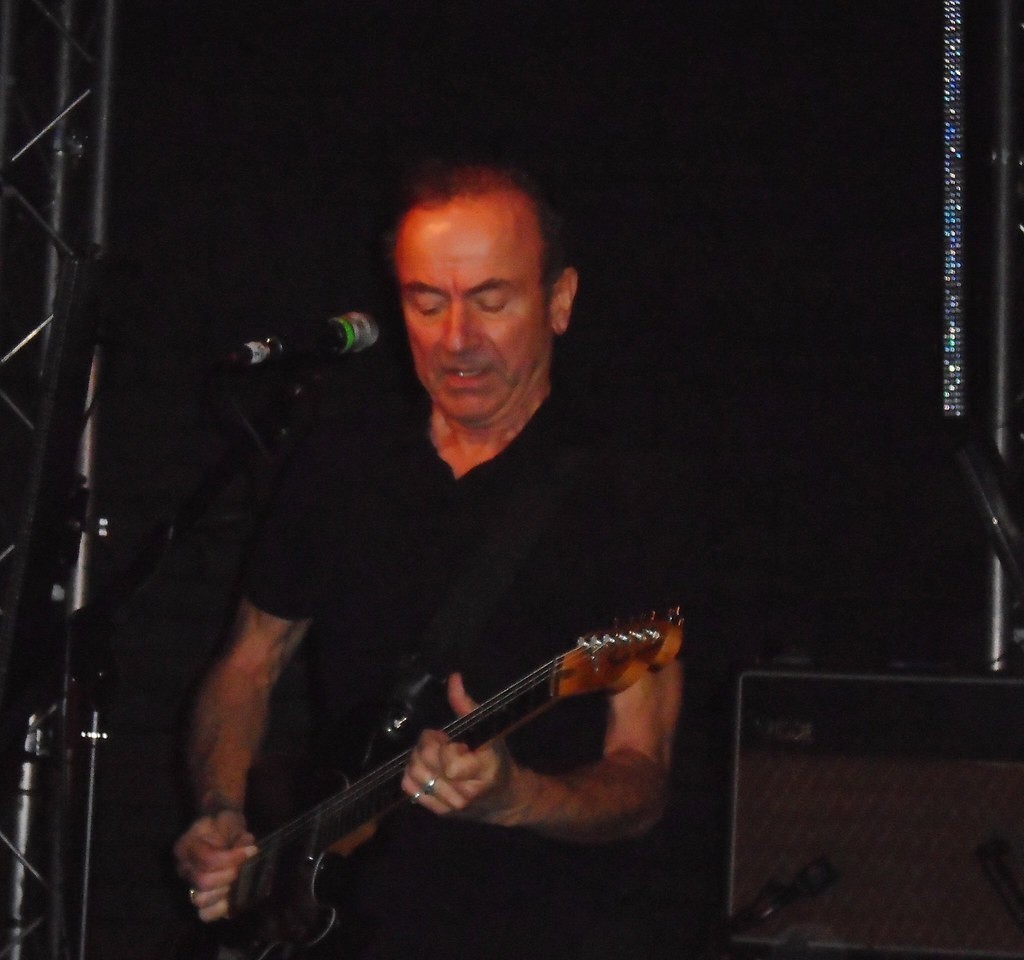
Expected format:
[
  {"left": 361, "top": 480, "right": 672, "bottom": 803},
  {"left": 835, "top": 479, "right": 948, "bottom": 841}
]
[{"left": 228, "top": 310, "right": 381, "bottom": 367}]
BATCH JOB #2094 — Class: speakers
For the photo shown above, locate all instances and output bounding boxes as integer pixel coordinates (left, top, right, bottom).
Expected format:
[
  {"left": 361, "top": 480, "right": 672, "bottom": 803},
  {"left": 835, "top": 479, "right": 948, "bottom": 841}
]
[{"left": 715, "top": 654, "right": 1024, "bottom": 960}]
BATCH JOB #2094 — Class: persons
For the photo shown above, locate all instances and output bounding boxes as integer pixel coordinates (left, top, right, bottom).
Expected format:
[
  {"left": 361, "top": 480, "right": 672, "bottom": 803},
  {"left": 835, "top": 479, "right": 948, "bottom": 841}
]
[{"left": 170, "top": 161, "right": 691, "bottom": 960}]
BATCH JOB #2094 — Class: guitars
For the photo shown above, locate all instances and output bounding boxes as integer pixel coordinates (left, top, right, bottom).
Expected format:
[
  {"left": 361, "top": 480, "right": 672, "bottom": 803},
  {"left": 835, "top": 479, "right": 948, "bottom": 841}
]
[{"left": 213, "top": 606, "right": 688, "bottom": 960}]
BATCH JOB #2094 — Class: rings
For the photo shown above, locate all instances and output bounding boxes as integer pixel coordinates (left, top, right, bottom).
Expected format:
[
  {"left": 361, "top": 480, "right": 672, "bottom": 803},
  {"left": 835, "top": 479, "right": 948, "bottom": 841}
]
[
  {"left": 423, "top": 776, "right": 436, "bottom": 793},
  {"left": 411, "top": 791, "right": 422, "bottom": 800},
  {"left": 189, "top": 889, "right": 196, "bottom": 903}
]
